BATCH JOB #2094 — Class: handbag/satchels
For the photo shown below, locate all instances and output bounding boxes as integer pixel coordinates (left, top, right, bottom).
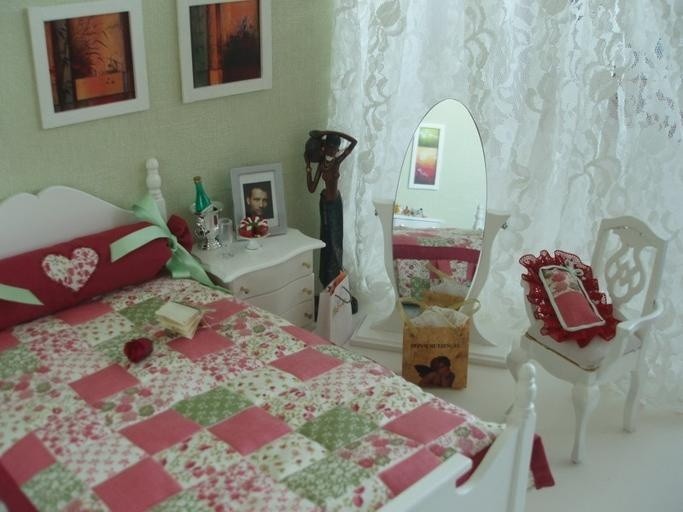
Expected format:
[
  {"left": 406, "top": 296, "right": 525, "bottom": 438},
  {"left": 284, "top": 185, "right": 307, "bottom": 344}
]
[
  {"left": 317, "top": 271, "right": 355, "bottom": 347},
  {"left": 396, "top": 296, "right": 481, "bottom": 388}
]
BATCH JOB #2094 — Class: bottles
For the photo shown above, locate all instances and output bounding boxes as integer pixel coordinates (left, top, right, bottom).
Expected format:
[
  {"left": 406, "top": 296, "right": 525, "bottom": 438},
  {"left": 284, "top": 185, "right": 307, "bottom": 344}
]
[{"left": 192, "top": 175, "right": 213, "bottom": 213}]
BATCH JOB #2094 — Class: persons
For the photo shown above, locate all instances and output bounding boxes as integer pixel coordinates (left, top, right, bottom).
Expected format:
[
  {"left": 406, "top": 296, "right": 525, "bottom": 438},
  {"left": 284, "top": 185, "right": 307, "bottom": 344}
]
[
  {"left": 303, "top": 129, "right": 359, "bottom": 286},
  {"left": 246, "top": 184, "right": 268, "bottom": 219},
  {"left": 418, "top": 355, "right": 455, "bottom": 387}
]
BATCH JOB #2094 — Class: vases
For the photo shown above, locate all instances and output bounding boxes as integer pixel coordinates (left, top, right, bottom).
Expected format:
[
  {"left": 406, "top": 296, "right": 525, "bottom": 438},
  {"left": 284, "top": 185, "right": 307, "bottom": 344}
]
[{"left": 235, "top": 233, "right": 270, "bottom": 251}]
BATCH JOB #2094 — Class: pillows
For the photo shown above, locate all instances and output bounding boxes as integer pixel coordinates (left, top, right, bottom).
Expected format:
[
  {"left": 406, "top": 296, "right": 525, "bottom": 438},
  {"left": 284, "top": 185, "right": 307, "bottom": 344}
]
[
  {"left": 516, "top": 248, "right": 623, "bottom": 349},
  {"left": 0, "top": 194, "right": 232, "bottom": 332}
]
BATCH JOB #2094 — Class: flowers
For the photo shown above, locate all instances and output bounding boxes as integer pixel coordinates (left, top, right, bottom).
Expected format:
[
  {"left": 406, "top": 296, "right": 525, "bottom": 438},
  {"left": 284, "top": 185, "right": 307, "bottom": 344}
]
[{"left": 237, "top": 216, "right": 268, "bottom": 238}]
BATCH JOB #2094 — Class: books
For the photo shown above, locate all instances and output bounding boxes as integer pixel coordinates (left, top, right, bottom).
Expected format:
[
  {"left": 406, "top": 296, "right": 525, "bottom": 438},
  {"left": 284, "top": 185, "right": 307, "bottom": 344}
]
[{"left": 155, "top": 300, "right": 216, "bottom": 341}]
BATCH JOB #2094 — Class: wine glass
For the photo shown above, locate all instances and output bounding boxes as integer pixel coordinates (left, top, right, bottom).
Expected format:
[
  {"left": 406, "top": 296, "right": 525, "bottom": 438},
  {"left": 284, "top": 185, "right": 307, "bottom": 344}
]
[{"left": 219, "top": 218, "right": 234, "bottom": 259}]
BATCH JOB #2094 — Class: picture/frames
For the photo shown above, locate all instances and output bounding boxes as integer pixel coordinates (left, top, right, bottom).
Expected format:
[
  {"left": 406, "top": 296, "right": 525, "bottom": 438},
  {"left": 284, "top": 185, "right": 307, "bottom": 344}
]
[
  {"left": 22, "top": 0, "right": 274, "bottom": 132},
  {"left": 229, "top": 162, "right": 287, "bottom": 240},
  {"left": 407, "top": 122, "right": 444, "bottom": 191}
]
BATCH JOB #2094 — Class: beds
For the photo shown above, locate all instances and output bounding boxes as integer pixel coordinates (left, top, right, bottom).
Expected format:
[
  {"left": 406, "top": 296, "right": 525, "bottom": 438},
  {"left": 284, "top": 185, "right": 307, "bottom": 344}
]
[
  {"left": 391, "top": 225, "right": 482, "bottom": 305},
  {"left": 0, "top": 183, "right": 541, "bottom": 512}
]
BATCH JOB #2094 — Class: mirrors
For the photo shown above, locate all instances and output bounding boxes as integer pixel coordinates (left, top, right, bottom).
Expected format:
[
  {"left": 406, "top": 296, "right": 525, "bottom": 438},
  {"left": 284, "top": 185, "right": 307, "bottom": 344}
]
[{"left": 389, "top": 95, "right": 488, "bottom": 325}]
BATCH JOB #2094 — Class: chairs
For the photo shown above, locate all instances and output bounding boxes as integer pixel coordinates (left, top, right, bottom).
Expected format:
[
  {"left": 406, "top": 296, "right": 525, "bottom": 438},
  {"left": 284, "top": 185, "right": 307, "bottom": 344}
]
[{"left": 504, "top": 214, "right": 668, "bottom": 465}]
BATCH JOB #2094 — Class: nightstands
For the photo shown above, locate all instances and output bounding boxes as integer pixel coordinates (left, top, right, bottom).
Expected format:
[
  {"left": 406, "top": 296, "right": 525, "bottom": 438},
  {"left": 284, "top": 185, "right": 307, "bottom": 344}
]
[{"left": 189, "top": 224, "right": 326, "bottom": 333}]
